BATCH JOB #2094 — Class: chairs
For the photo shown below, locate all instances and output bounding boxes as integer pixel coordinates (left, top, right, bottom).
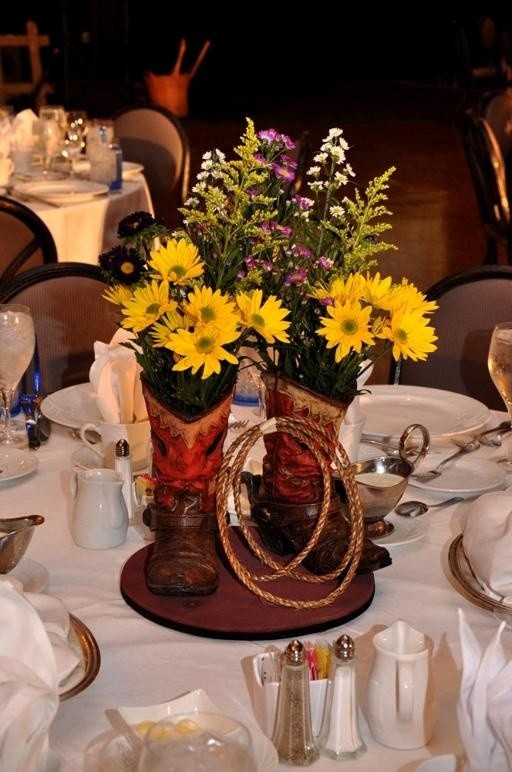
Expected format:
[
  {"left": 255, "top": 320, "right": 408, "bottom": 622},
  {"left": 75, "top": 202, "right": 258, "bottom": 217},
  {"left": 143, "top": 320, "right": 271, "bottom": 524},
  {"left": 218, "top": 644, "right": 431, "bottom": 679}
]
[
  {"left": 0, "top": 198, "right": 57, "bottom": 278},
  {"left": 111, "top": 103, "right": 190, "bottom": 226},
  {"left": 0, "top": 263, "right": 132, "bottom": 397}
]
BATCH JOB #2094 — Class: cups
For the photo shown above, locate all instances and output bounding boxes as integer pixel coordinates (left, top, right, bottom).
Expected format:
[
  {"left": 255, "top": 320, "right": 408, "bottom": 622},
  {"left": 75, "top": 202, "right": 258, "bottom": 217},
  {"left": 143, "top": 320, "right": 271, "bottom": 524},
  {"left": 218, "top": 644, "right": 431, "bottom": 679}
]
[
  {"left": 73, "top": 421, "right": 155, "bottom": 462},
  {"left": 138, "top": 716, "right": 252, "bottom": 770},
  {"left": 264, "top": 679, "right": 332, "bottom": 742},
  {"left": 486, "top": 322, "right": 511, "bottom": 419},
  {"left": 0, "top": 103, "right": 119, "bottom": 184},
  {"left": 352, "top": 425, "right": 429, "bottom": 522}
]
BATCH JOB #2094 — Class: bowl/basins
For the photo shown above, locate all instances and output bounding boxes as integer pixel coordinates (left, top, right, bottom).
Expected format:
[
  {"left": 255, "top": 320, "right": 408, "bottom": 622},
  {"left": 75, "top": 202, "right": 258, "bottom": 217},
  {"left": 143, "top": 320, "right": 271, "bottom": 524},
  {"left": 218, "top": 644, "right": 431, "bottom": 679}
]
[{"left": 0, "top": 516, "right": 43, "bottom": 572}]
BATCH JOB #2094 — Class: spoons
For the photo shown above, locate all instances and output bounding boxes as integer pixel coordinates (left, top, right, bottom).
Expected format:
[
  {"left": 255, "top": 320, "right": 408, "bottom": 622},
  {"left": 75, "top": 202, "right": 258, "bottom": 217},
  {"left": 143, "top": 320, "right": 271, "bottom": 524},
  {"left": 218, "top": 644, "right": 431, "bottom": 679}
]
[{"left": 396, "top": 495, "right": 463, "bottom": 516}]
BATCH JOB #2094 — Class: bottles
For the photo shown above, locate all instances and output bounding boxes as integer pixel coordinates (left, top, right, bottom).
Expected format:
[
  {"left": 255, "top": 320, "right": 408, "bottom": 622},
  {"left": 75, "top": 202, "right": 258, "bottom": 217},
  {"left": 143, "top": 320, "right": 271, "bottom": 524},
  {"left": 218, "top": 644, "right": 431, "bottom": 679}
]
[
  {"left": 325, "top": 635, "right": 364, "bottom": 757},
  {"left": 113, "top": 438, "right": 142, "bottom": 519},
  {"left": 274, "top": 639, "right": 319, "bottom": 766}
]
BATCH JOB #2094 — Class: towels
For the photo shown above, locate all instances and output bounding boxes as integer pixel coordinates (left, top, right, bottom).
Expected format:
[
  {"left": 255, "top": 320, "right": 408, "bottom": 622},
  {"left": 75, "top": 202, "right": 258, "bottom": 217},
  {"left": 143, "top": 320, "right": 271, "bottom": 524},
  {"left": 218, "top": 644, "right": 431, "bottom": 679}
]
[
  {"left": 462, "top": 491, "right": 512, "bottom": 605},
  {"left": 0, "top": 575, "right": 80, "bottom": 771},
  {"left": 456, "top": 607, "right": 512, "bottom": 772},
  {"left": 89, "top": 341, "right": 144, "bottom": 424},
  {"left": 9, "top": 109, "right": 39, "bottom": 149}
]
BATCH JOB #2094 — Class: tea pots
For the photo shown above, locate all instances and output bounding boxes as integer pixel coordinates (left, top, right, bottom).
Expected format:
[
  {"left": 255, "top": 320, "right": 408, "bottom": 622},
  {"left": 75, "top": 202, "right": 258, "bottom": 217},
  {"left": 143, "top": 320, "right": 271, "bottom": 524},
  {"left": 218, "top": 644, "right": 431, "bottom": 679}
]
[
  {"left": 67, "top": 469, "right": 130, "bottom": 549},
  {"left": 364, "top": 618, "right": 441, "bottom": 750}
]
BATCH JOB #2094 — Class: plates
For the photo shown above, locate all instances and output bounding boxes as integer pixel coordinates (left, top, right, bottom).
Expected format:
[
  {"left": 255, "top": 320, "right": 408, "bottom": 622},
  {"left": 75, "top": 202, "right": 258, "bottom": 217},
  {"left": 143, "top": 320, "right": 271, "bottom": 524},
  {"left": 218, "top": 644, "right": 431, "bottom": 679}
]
[
  {"left": 416, "top": 749, "right": 471, "bottom": 770},
  {"left": 40, "top": 382, "right": 100, "bottom": 428},
  {"left": 118, "top": 686, "right": 241, "bottom": 748},
  {"left": 70, "top": 161, "right": 143, "bottom": 181},
  {"left": 366, "top": 514, "right": 423, "bottom": 549},
  {"left": 357, "top": 438, "right": 385, "bottom": 464},
  {"left": 445, "top": 533, "right": 512, "bottom": 617},
  {"left": 15, "top": 179, "right": 108, "bottom": 203},
  {"left": 70, "top": 441, "right": 155, "bottom": 470},
  {"left": 0, "top": 450, "right": 38, "bottom": 485},
  {"left": 410, "top": 451, "right": 504, "bottom": 494},
  {"left": 31, "top": 612, "right": 99, "bottom": 698},
  {"left": 0, "top": 558, "right": 49, "bottom": 596},
  {"left": 357, "top": 382, "right": 486, "bottom": 446}
]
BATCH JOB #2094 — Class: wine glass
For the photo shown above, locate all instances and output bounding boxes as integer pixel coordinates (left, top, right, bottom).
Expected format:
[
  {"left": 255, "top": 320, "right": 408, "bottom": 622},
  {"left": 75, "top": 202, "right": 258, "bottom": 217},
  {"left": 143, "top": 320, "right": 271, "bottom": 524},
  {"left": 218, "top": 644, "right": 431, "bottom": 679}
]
[{"left": 0, "top": 302, "right": 36, "bottom": 444}]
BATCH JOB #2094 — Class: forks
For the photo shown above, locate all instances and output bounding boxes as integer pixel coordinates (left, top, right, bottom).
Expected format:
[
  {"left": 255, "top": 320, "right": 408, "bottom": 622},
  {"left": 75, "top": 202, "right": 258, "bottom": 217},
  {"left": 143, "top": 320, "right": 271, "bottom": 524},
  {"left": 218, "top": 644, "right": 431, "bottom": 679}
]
[
  {"left": 446, "top": 420, "right": 511, "bottom": 450},
  {"left": 411, "top": 441, "right": 483, "bottom": 481}
]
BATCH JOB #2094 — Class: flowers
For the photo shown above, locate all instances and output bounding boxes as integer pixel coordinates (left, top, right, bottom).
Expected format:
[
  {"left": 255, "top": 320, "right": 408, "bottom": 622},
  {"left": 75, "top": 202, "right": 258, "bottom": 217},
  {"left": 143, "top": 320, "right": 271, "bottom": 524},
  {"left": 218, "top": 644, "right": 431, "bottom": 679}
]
[
  {"left": 96, "top": 116, "right": 292, "bottom": 419},
  {"left": 193, "top": 125, "right": 440, "bottom": 403}
]
